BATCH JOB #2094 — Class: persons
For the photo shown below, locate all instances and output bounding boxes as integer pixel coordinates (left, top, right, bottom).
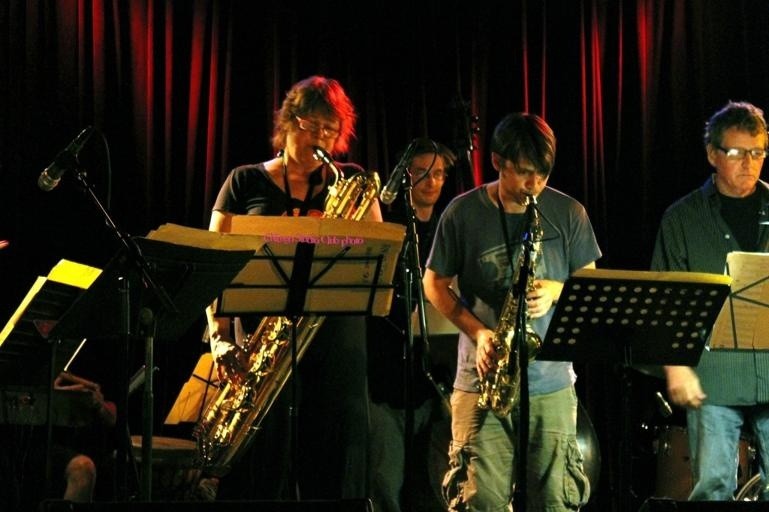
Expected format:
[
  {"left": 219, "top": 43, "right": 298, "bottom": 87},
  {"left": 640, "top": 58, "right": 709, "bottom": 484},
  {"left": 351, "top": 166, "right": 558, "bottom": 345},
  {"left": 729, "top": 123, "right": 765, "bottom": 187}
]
[
  {"left": 367, "top": 137, "right": 457, "bottom": 512},
  {"left": 649, "top": 101, "right": 769, "bottom": 503},
  {"left": 204, "top": 76, "right": 383, "bottom": 500},
  {"left": 34, "top": 317, "right": 124, "bottom": 504},
  {"left": 421, "top": 110, "right": 604, "bottom": 512}
]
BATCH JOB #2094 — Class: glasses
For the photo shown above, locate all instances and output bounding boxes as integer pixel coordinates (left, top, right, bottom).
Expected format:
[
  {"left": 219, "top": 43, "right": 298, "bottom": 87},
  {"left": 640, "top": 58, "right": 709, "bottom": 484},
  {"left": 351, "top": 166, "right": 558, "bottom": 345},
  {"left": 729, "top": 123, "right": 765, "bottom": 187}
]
[
  {"left": 294, "top": 114, "right": 341, "bottom": 140},
  {"left": 714, "top": 142, "right": 769, "bottom": 162}
]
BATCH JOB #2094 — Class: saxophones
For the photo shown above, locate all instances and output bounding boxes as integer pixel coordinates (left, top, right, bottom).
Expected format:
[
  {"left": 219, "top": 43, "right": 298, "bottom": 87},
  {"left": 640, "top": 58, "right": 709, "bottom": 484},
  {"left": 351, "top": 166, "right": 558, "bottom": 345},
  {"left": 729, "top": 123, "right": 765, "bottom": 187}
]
[
  {"left": 476, "top": 197, "right": 545, "bottom": 420},
  {"left": 192, "top": 145, "right": 381, "bottom": 475}
]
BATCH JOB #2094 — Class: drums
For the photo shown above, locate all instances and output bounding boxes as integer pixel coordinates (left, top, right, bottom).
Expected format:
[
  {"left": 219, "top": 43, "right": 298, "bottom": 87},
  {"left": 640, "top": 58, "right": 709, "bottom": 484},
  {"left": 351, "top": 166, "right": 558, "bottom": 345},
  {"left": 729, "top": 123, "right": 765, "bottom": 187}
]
[{"left": 121, "top": 435, "right": 196, "bottom": 498}]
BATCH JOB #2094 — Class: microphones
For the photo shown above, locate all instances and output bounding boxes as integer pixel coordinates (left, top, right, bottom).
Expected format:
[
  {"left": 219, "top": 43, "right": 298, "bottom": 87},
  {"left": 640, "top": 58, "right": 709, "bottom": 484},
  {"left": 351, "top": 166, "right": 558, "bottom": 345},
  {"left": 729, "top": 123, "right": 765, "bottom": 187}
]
[
  {"left": 510, "top": 204, "right": 536, "bottom": 250},
  {"left": 37, "top": 124, "right": 93, "bottom": 192},
  {"left": 381, "top": 144, "right": 417, "bottom": 205}
]
